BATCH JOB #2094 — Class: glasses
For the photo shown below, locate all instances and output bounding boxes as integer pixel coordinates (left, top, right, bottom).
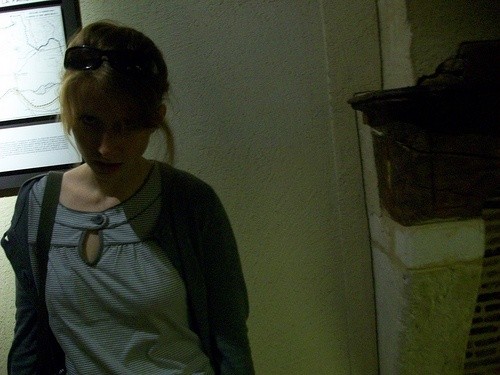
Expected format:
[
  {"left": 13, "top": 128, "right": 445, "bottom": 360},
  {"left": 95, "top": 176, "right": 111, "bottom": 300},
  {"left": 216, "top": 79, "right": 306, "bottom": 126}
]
[{"left": 63, "top": 45, "right": 155, "bottom": 79}]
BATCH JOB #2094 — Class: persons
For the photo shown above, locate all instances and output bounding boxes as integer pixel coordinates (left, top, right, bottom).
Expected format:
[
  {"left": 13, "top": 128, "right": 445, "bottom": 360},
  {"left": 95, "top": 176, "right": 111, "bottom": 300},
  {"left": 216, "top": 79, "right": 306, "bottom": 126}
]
[{"left": 0, "top": 17, "right": 255, "bottom": 371}]
